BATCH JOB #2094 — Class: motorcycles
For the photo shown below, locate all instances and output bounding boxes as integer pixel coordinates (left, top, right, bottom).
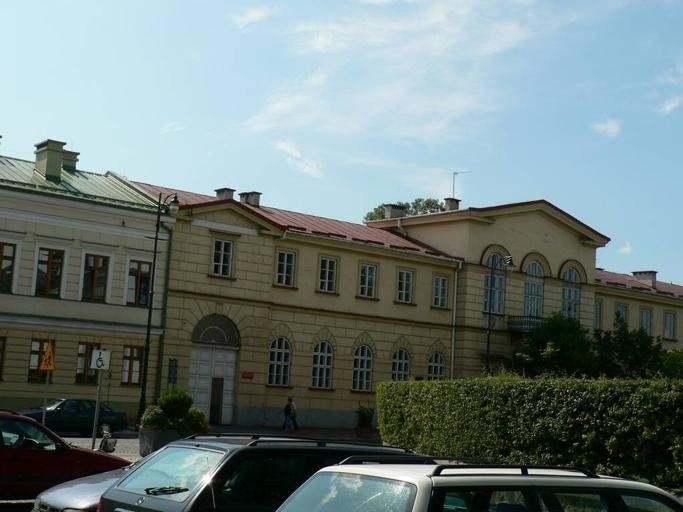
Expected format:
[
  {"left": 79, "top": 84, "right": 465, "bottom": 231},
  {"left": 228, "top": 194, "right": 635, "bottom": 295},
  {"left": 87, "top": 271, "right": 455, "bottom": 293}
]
[{"left": 96, "top": 430, "right": 117, "bottom": 453}]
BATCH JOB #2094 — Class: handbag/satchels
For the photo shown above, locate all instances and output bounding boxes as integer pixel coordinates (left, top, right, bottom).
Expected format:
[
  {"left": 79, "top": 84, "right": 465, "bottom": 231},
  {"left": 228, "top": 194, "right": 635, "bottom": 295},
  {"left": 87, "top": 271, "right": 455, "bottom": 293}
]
[{"left": 292, "top": 409, "right": 297, "bottom": 416}]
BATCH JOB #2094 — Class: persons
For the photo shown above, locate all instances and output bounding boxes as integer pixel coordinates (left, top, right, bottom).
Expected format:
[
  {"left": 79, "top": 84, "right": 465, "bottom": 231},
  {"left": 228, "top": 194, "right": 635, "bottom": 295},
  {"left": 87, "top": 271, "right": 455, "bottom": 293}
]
[
  {"left": 277, "top": 397, "right": 295, "bottom": 433},
  {"left": 290, "top": 397, "right": 299, "bottom": 431}
]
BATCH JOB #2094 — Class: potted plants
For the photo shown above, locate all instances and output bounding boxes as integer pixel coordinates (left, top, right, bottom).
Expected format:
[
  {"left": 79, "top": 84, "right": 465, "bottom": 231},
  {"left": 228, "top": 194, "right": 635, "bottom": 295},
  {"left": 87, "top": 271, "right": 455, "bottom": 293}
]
[{"left": 353, "top": 401, "right": 375, "bottom": 439}]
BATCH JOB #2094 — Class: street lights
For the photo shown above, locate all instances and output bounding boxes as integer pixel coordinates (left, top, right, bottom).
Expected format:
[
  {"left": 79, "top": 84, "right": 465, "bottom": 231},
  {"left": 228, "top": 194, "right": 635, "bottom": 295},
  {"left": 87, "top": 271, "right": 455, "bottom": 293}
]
[
  {"left": 482, "top": 252, "right": 516, "bottom": 380},
  {"left": 132, "top": 187, "right": 181, "bottom": 433}
]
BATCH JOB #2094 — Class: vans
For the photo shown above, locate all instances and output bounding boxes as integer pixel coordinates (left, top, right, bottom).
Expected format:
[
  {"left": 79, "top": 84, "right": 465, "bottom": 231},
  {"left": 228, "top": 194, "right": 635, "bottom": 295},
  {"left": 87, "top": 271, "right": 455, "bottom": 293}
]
[
  {"left": 277, "top": 457, "right": 681, "bottom": 511},
  {"left": 101, "top": 431, "right": 460, "bottom": 512}
]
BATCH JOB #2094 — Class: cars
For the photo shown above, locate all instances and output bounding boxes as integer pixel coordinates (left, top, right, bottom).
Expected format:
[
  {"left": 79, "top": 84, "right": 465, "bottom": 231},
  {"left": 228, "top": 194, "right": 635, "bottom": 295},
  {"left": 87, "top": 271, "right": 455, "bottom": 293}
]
[
  {"left": 19, "top": 396, "right": 130, "bottom": 437},
  {"left": 30, "top": 456, "right": 159, "bottom": 509},
  {"left": 0, "top": 409, "right": 131, "bottom": 511}
]
